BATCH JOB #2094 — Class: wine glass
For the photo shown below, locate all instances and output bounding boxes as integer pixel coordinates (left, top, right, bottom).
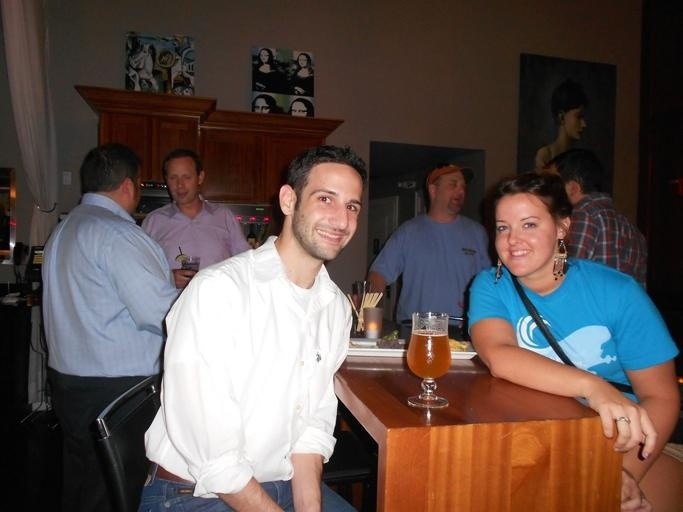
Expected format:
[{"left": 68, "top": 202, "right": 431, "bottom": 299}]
[{"left": 406, "top": 312, "right": 451, "bottom": 409}]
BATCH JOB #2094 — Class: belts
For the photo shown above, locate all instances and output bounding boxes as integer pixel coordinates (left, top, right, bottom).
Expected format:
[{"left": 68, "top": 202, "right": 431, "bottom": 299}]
[{"left": 155, "top": 466, "right": 193, "bottom": 484}]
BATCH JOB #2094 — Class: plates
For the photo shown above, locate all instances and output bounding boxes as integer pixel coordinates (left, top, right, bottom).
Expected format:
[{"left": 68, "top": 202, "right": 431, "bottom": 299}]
[
  {"left": 345, "top": 337, "right": 406, "bottom": 358},
  {"left": 451, "top": 340, "right": 478, "bottom": 360}
]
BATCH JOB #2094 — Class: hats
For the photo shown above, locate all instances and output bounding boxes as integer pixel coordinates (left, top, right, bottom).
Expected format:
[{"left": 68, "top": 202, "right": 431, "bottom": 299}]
[{"left": 427, "top": 162, "right": 474, "bottom": 184}]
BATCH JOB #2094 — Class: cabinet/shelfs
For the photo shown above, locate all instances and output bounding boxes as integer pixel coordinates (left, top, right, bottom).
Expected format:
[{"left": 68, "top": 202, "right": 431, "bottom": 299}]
[
  {"left": 76, "top": 85, "right": 216, "bottom": 184},
  {"left": 199, "top": 111, "right": 345, "bottom": 203}
]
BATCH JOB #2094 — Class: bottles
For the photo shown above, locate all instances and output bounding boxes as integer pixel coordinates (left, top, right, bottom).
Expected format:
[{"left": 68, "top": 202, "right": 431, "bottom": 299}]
[{"left": 246, "top": 224, "right": 256, "bottom": 247}]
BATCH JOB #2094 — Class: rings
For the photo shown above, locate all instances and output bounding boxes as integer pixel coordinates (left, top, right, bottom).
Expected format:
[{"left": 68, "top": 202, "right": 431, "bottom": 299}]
[{"left": 617, "top": 417, "right": 631, "bottom": 424}]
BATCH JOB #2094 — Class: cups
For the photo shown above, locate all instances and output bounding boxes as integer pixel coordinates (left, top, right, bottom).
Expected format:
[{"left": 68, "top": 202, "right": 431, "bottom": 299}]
[
  {"left": 181, "top": 256, "right": 200, "bottom": 275},
  {"left": 363, "top": 307, "right": 384, "bottom": 337}
]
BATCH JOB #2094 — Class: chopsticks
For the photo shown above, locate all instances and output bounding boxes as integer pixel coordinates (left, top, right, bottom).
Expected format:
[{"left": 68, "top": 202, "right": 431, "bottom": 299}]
[{"left": 346, "top": 292, "right": 383, "bottom": 331}]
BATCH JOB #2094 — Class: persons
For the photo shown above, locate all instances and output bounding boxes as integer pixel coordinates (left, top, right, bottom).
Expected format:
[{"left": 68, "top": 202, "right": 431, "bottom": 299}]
[
  {"left": 137, "top": 144, "right": 365, "bottom": 511},
  {"left": 542, "top": 151, "right": 651, "bottom": 293},
  {"left": 288, "top": 97, "right": 315, "bottom": 119},
  {"left": 534, "top": 79, "right": 604, "bottom": 185},
  {"left": 365, "top": 161, "right": 493, "bottom": 341},
  {"left": 463, "top": 170, "right": 682, "bottom": 510},
  {"left": 252, "top": 94, "right": 289, "bottom": 116},
  {"left": 40, "top": 142, "right": 179, "bottom": 511},
  {"left": 286, "top": 51, "right": 315, "bottom": 97},
  {"left": 143, "top": 150, "right": 257, "bottom": 290},
  {"left": 253, "top": 44, "right": 277, "bottom": 93}
]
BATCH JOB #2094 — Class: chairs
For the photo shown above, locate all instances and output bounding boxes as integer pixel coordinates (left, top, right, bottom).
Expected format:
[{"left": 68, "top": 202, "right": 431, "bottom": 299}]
[
  {"left": 323, "top": 432, "right": 376, "bottom": 512},
  {"left": 96, "top": 375, "right": 161, "bottom": 512}
]
